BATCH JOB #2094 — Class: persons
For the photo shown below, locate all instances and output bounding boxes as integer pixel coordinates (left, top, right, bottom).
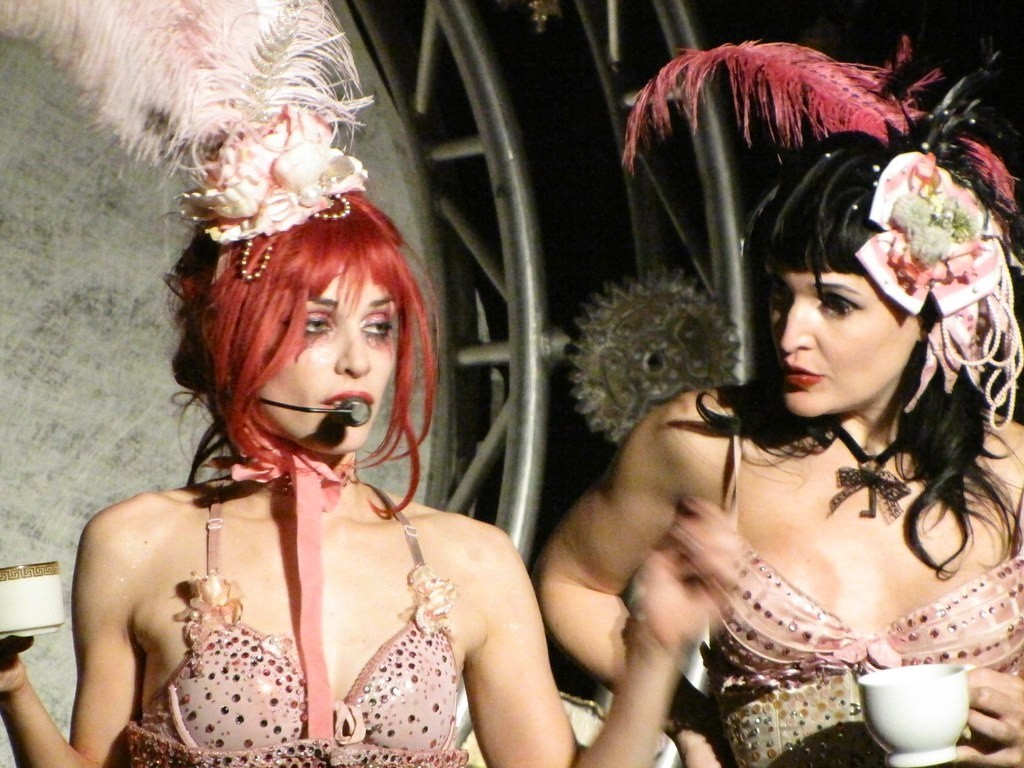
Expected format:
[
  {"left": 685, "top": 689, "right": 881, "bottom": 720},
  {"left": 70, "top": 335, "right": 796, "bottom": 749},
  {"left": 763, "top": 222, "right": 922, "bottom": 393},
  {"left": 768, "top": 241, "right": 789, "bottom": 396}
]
[
  {"left": 527, "top": 129, "right": 1024, "bottom": 768},
  {"left": 1, "top": 187, "right": 740, "bottom": 767}
]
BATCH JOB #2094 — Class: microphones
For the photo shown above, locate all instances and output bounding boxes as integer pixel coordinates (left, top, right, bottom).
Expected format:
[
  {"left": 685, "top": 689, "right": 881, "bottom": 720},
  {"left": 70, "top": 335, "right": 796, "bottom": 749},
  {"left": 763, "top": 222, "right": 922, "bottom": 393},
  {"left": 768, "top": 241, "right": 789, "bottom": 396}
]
[{"left": 261, "top": 397, "right": 371, "bottom": 427}]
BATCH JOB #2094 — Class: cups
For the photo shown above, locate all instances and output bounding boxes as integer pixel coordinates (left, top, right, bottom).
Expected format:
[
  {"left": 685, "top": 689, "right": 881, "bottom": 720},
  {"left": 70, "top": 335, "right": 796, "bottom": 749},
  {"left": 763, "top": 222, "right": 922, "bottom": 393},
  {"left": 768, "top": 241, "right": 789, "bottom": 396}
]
[
  {"left": 0, "top": 561, "right": 65, "bottom": 641},
  {"left": 856, "top": 663, "right": 969, "bottom": 767}
]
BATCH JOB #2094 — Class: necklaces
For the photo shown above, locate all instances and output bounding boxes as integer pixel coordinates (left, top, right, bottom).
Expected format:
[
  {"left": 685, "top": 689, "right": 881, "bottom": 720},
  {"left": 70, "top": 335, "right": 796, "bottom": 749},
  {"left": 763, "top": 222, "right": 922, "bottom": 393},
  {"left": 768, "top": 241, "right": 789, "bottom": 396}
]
[{"left": 831, "top": 423, "right": 914, "bottom": 522}]
